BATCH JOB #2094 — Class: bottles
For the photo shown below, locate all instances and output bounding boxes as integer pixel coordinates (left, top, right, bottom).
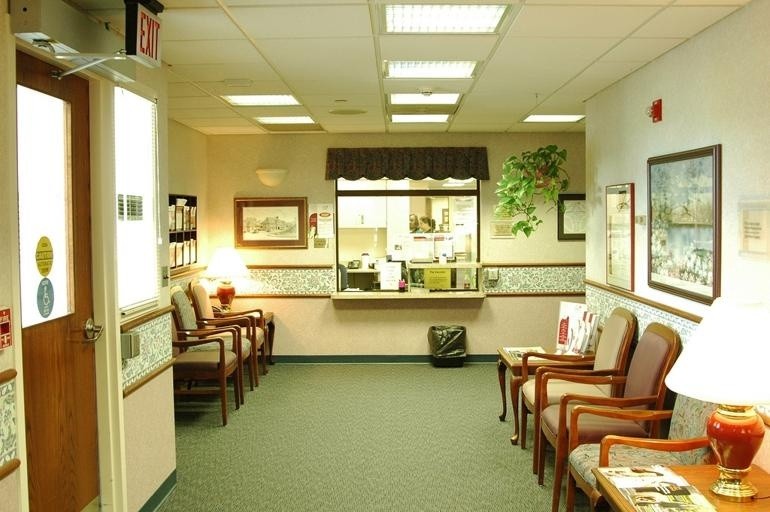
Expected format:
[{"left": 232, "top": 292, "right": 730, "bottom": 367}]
[{"left": 464, "top": 274, "right": 470, "bottom": 288}]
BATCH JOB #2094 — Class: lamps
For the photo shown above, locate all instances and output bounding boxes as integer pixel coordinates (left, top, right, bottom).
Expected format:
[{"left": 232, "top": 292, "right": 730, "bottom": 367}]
[
  {"left": 256, "top": 168, "right": 286, "bottom": 187},
  {"left": 202, "top": 247, "right": 252, "bottom": 311},
  {"left": 659, "top": 293, "right": 770, "bottom": 502}
]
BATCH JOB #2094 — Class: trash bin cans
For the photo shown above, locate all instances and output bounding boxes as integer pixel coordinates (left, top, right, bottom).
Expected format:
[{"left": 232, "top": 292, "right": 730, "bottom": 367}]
[{"left": 427, "top": 325, "right": 467, "bottom": 368}]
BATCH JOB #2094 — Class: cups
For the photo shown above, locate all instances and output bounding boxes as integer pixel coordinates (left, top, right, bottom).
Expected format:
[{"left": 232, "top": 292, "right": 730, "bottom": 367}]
[{"left": 399, "top": 281, "right": 406, "bottom": 292}]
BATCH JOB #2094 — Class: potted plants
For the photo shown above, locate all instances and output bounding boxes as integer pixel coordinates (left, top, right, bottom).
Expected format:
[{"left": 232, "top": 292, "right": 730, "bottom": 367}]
[{"left": 496, "top": 143, "right": 571, "bottom": 239}]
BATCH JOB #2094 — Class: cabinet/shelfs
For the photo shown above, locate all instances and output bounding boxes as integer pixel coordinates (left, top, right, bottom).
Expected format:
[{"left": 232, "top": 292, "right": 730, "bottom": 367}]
[
  {"left": 337, "top": 179, "right": 386, "bottom": 228},
  {"left": 386, "top": 177, "right": 411, "bottom": 262},
  {"left": 169, "top": 193, "right": 199, "bottom": 271}
]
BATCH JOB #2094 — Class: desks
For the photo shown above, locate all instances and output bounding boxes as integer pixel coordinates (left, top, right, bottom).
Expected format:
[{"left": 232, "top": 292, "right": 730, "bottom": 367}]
[
  {"left": 585, "top": 457, "right": 769, "bottom": 512},
  {"left": 346, "top": 268, "right": 376, "bottom": 275},
  {"left": 495, "top": 343, "right": 595, "bottom": 445},
  {"left": 229, "top": 308, "right": 276, "bottom": 367}
]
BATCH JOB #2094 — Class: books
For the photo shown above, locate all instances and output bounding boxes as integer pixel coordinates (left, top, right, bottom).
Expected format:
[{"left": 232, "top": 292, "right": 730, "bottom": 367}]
[
  {"left": 503, "top": 299, "right": 600, "bottom": 363},
  {"left": 597, "top": 463, "right": 716, "bottom": 512}
]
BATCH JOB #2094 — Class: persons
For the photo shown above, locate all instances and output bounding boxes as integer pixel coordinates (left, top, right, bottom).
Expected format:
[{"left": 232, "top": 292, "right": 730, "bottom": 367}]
[
  {"left": 418, "top": 215, "right": 437, "bottom": 233},
  {"left": 410, "top": 213, "right": 423, "bottom": 233}
]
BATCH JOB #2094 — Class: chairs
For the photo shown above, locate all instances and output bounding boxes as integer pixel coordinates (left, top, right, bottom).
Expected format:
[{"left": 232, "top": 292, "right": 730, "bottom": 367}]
[
  {"left": 520, "top": 304, "right": 640, "bottom": 474},
  {"left": 169, "top": 278, "right": 267, "bottom": 427},
  {"left": 536, "top": 320, "right": 683, "bottom": 510},
  {"left": 565, "top": 387, "right": 720, "bottom": 512}
]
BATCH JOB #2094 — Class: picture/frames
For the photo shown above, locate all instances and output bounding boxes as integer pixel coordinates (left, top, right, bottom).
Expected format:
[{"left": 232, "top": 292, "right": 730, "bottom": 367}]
[
  {"left": 645, "top": 142, "right": 723, "bottom": 308},
  {"left": 234, "top": 196, "right": 308, "bottom": 250},
  {"left": 489, "top": 219, "right": 516, "bottom": 240},
  {"left": 557, "top": 193, "right": 585, "bottom": 241}
]
